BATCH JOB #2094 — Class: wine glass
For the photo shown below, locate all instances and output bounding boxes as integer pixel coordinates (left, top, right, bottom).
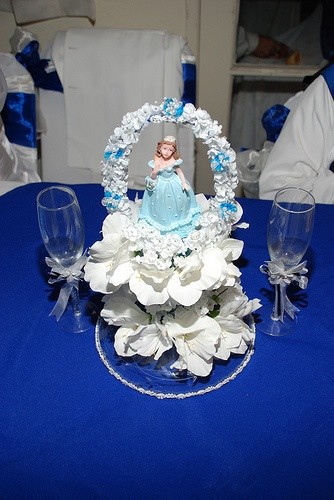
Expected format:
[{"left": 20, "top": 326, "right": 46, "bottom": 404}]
[
  {"left": 36, "top": 186, "right": 100, "bottom": 334},
  {"left": 253, "top": 183, "right": 315, "bottom": 338}
]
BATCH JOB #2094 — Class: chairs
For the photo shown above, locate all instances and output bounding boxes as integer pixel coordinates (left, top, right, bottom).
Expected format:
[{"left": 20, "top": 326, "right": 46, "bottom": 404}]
[{"left": 36, "top": 28, "right": 197, "bottom": 198}]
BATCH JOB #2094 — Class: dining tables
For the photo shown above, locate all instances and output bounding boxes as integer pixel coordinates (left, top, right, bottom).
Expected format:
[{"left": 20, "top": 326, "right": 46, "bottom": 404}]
[{"left": 0, "top": 181, "right": 334, "bottom": 500}]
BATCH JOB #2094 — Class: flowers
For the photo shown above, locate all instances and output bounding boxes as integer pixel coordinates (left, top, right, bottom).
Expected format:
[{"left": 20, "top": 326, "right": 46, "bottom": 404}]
[{"left": 83, "top": 97, "right": 262, "bottom": 377}]
